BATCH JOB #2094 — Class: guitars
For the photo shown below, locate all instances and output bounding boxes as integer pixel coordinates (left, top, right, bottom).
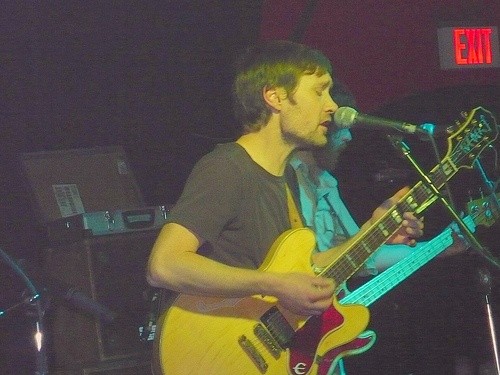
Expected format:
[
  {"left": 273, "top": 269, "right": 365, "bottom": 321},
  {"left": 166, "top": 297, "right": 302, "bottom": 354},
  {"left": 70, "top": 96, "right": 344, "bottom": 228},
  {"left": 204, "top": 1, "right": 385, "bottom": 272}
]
[
  {"left": 315, "top": 191, "right": 500, "bottom": 375},
  {"left": 151, "top": 106, "right": 500, "bottom": 375}
]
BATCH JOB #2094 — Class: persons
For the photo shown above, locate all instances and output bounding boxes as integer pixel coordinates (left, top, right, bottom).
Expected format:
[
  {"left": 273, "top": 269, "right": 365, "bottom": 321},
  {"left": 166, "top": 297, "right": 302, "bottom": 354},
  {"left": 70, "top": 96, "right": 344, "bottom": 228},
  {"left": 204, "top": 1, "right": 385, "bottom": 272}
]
[
  {"left": 146, "top": 42, "right": 424, "bottom": 375},
  {"left": 289, "top": 84, "right": 472, "bottom": 375},
  {"left": 0, "top": 219, "right": 138, "bottom": 375}
]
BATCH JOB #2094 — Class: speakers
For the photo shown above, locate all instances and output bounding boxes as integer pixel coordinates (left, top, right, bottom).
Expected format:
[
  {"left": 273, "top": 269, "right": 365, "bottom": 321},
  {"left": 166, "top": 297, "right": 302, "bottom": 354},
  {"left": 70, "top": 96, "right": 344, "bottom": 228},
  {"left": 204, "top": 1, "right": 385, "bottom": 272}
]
[{"left": 36, "top": 230, "right": 178, "bottom": 367}]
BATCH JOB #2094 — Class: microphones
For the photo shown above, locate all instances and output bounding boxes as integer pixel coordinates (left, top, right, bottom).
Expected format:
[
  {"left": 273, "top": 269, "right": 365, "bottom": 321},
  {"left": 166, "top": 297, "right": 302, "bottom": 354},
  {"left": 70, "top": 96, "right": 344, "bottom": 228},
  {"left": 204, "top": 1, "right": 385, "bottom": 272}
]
[
  {"left": 334, "top": 107, "right": 428, "bottom": 135},
  {"left": 419, "top": 123, "right": 463, "bottom": 141}
]
